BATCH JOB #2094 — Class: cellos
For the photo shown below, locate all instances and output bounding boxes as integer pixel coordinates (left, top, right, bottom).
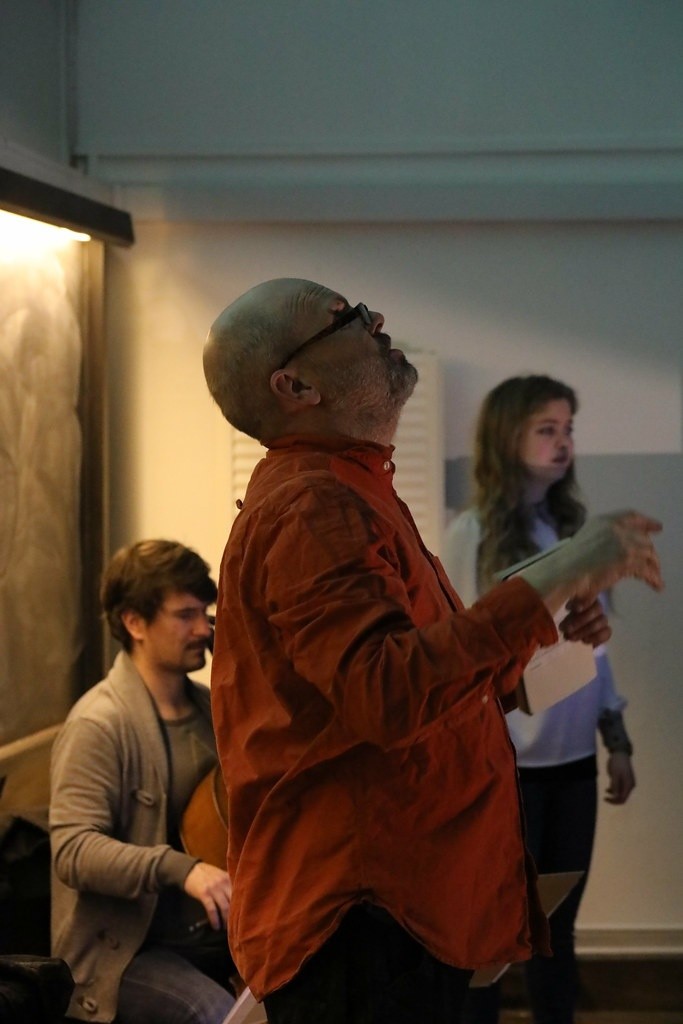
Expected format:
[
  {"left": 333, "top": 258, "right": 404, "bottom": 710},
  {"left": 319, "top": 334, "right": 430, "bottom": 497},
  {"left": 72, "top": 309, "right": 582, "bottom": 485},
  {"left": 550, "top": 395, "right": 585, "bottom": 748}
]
[{"left": 178, "top": 614, "right": 230, "bottom": 875}]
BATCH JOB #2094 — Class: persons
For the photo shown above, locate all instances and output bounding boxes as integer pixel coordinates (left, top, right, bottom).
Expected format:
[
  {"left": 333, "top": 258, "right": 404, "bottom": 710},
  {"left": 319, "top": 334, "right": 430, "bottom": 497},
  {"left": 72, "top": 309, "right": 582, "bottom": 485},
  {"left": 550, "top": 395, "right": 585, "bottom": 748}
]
[
  {"left": 438, "top": 373, "right": 638, "bottom": 1024},
  {"left": 204, "top": 276, "right": 664, "bottom": 1024},
  {"left": 49, "top": 541, "right": 248, "bottom": 1024}
]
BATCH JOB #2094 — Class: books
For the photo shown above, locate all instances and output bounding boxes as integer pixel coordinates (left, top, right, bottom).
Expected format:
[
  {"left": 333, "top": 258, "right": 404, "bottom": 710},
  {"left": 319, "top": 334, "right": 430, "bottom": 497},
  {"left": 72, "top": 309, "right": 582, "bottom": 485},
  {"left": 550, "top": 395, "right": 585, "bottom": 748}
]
[{"left": 491, "top": 538, "right": 597, "bottom": 716}]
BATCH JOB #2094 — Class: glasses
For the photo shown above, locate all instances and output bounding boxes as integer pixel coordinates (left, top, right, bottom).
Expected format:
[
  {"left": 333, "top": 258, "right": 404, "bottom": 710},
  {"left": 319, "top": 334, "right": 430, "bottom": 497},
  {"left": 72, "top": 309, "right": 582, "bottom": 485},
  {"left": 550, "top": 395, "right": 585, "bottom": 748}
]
[{"left": 276, "top": 301, "right": 373, "bottom": 369}]
